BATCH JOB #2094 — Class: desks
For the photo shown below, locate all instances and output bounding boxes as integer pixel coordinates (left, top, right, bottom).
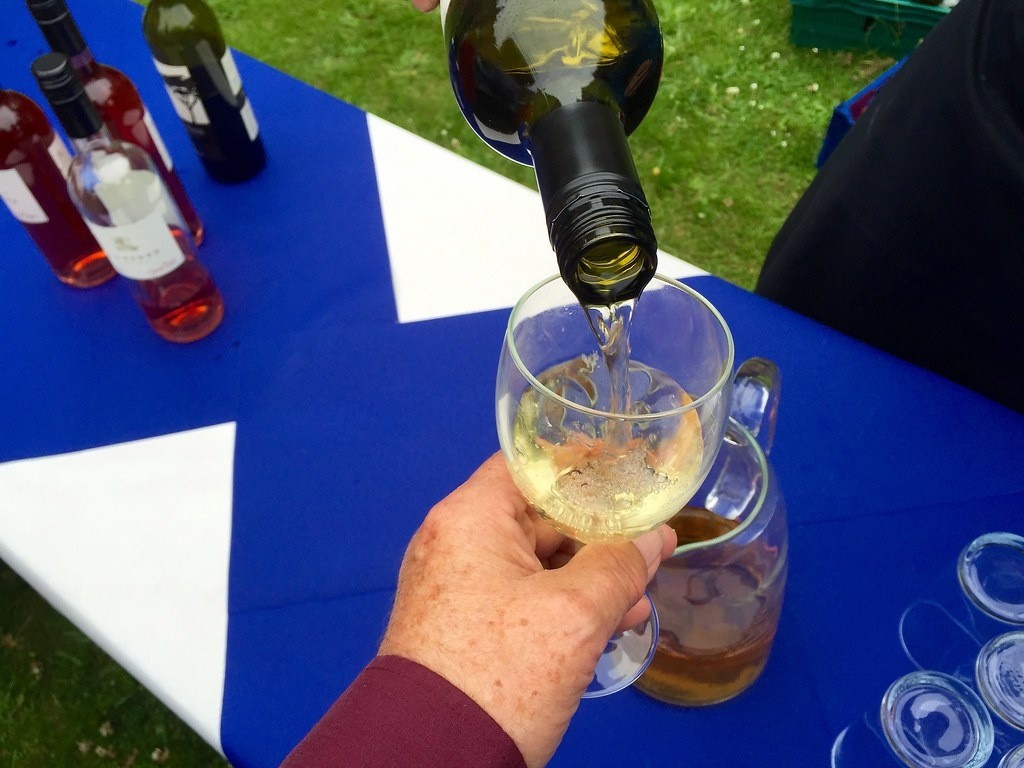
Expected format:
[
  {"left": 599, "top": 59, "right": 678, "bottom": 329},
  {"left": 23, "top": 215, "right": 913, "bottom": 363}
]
[{"left": 0, "top": 0, "right": 1024, "bottom": 768}]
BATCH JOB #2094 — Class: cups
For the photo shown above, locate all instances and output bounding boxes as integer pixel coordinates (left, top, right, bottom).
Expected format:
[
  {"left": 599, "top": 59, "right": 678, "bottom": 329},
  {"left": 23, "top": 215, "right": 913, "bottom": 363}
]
[
  {"left": 953, "top": 632, "right": 1024, "bottom": 742},
  {"left": 831, "top": 671, "right": 995, "bottom": 768},
  {"left": 991, "top": 742, "right": 1024, "bottom": 768},
  {"left": 625, "top": 358, "right": 787, "bottom": 705},
  {"left": 900, "top": 532, "right": 1024, "bottom": 680}
]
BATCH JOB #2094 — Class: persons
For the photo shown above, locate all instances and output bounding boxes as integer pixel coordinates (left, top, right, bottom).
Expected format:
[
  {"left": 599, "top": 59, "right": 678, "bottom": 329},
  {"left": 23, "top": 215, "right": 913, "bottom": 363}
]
[
  {"left": 275, "top": 436, "right": 679, "bottom": 768},
  {"left": 413, "top": 0, "right": 1024, "bottom": 424}
]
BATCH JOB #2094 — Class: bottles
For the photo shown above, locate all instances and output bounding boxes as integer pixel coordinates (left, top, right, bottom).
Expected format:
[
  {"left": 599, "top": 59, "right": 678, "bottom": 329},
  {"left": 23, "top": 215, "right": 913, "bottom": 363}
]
[
  {"left": 0, "top": 90, "right": 117, "bottom": 286},
  {"left": 33, "top": 55, "right": 225, "bottom": 346},
  {"left": 26, "top": 0, "right": 203, "bottom": 246},
  {"left": 436, "top": 0, "right": 665, "bottom": 306},
  {"left": 139, "top": 0, "right": 267, "bottom": 184}
]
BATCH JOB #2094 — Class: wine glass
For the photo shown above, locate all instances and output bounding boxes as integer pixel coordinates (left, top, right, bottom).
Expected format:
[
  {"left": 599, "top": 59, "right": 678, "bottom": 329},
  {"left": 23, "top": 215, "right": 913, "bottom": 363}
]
[{"left": 496, "top": 268, "right": 738, "bottom": 699}]
